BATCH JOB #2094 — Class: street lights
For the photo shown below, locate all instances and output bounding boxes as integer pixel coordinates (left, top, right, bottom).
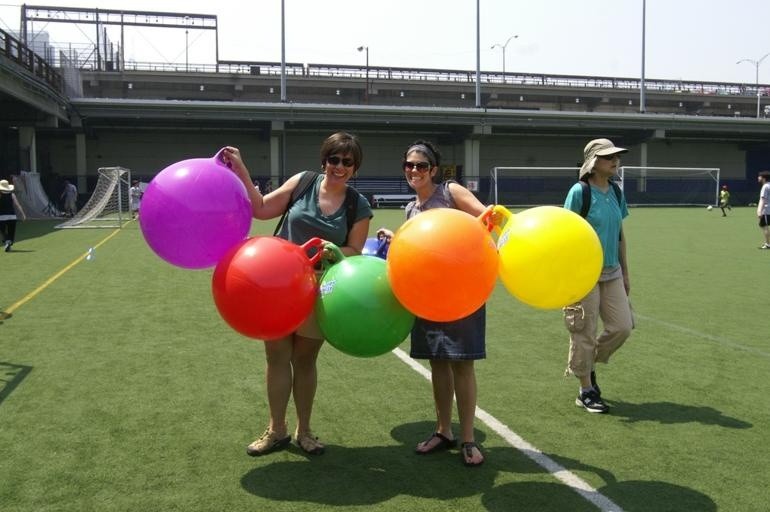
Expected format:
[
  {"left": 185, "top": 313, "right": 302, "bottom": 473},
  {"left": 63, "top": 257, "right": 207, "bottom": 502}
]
[
  {"left": 735, "top": 51, "right": 769, "bottom": 85},
  {"left": 357, "top": 45, "right": 369, "bottom": 104},
  {"left": 489, "top": 35, "right": 519, "bottom": 83}
]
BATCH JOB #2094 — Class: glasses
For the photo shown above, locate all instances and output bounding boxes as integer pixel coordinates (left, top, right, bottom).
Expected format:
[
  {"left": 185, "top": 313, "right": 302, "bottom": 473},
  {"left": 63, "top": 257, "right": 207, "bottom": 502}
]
[
  {"left": 327, "top": 155, "right": 355, "bottom": 167},
  {"left": 404, "top": 161, "right": 433, "bottom": 175},
  {"left": 598, "top": 155, "right": 621, "bottom": 160}
]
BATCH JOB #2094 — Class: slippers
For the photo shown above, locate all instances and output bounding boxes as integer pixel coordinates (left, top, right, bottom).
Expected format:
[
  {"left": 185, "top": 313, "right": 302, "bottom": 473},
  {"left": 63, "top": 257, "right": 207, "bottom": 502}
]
[
  {"left": 415, "top": 432, "right": 457, "bottom": 455},
  {"left": 461, "top": 441, "right": 485, "bottom": 467}
]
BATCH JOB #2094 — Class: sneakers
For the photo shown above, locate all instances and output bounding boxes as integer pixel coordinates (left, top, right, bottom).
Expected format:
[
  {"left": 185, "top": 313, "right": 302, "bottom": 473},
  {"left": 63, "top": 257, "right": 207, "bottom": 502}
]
[
  {"left": 575, "top": 371, "right": 609, "bottom": 413},
  {"left": 5, "top": 240, "right": 12, "bottom": 252},
  {"left": 762, "top": 243, "right": 770, "bottom": 249}
]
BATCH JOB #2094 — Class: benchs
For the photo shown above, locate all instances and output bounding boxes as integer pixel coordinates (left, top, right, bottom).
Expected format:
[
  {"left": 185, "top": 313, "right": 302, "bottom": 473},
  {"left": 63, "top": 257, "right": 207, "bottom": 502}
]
[{"left": 372, "top": 195, "right": 417, "bottom": 209}]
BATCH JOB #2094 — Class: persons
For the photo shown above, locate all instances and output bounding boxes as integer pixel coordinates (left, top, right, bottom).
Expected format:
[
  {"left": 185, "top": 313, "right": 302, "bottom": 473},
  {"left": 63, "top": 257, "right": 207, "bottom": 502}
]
[
  {"left": 222, "top": 132, "right": 374, "bottom": 456},
  {"left": 379, "top": 137, "right": 504, "bottom": 467},
  {"left": 60, "top": 181, "right": 77, "bottom": 217},
  {"left": 254, "top": 180, "right": 260, "bottom": 191},
  {"left": 129, "top": 180, "right": 142, "bottom": 218},
  {"left": 757, "top": 170, "right": 770, "bottom": 249},
  {"left": 719, "top": 185, "right": 731, "bottom": 216},
  {"left": 0, "top": 179, "right": 25, "bottom": 251},
  {"left": 561, "top": 138, "right": 633, "bottom": 414}
]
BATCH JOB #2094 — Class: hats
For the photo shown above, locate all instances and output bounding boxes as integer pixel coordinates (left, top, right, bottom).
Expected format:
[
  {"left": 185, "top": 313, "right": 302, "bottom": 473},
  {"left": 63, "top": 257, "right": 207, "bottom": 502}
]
[
  {"left": 578, "top": 138, "right": 630, "bottom": 181},
  {"left": 0, "top": 180, "right": 15, "bottom": 192}
]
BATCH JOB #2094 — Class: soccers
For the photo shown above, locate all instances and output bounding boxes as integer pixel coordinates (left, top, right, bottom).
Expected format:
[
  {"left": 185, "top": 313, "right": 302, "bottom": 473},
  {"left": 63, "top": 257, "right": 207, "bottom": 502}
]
[{"left": 707, "top": 205, "right": 712, "bottom": 210}]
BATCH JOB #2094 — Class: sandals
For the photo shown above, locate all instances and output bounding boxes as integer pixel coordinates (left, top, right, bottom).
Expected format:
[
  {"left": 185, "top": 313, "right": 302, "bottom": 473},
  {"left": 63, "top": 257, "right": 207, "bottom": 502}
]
[
  {"left": 294, "top": 427, "right": 326, "bottom": 456},
  {"left": 246, "top": 421, "right": 291, "bottom": 456}
]
